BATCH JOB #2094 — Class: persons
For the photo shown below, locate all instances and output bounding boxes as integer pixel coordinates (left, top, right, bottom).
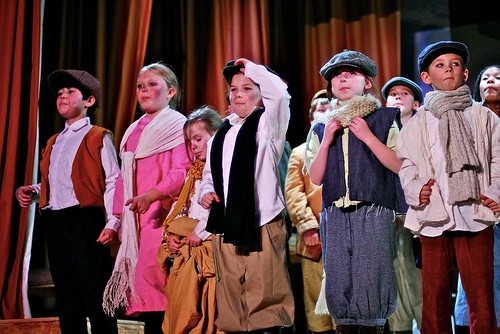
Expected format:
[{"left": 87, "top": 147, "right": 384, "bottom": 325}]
[
  {"left": 113, "top": 63, "right": 192, "bottom": 334},
  {"left": 15, "top": 69, "right": 121, "bottom": 334},
  {"left": 198, "top": 58, "right": 295, "bottom": 334},
  {"left": 226, "top": 65, "right": 500, "bottom": 334},
  {"left": 394, "top": 40, "right": 500, "bottom": 334},
  {"left": 156, "top": 107, "right": 224, "bottom": 334},
  {"left": 305, "top": 49, "right": 408, "bottom": 334}
]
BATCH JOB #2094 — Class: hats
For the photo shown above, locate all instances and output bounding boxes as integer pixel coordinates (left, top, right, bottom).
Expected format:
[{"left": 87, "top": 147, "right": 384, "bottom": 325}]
[
  {"left": 381, "top": 76, "right": 423, "bottom": 105},
  {"left": 418, "top": 41, "right": 469, "bottom": 75},
  {"left": 48, "top": 69, "right": 99, "bottom": 105},
  {"left": 222, "top": 60, "right": 278, "bottom": 87},
  {"left": 319, "top": 49, "right": 377, "bottom": 81},
  {"left": 312, "top": 88, "right": 327, "bottom": 99}
]
